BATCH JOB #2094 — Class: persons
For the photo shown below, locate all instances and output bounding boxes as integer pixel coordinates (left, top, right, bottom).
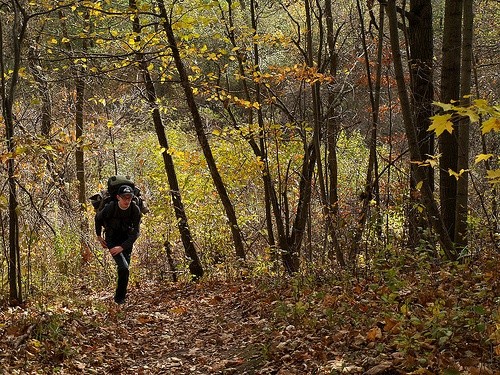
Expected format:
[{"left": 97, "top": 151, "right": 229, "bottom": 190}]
[{"left": 95, "top": 186, "right": 144, "bottom": 312}]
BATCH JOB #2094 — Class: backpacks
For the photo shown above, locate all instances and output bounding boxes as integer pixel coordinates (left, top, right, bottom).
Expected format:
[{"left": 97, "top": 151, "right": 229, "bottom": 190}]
[{"left": 108, "top": 174, "right": 136, "bottom": 199}]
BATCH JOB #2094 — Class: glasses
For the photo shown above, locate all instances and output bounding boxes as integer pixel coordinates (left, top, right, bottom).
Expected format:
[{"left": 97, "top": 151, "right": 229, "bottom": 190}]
[{"left": 119, "top": 195, "right": 133, "bottom": 200}]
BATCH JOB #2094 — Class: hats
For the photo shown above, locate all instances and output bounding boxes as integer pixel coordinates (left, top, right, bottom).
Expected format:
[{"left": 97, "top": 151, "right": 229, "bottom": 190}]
[{"left": 118, "top": 185, "right": 134, "bottom": 198}]
